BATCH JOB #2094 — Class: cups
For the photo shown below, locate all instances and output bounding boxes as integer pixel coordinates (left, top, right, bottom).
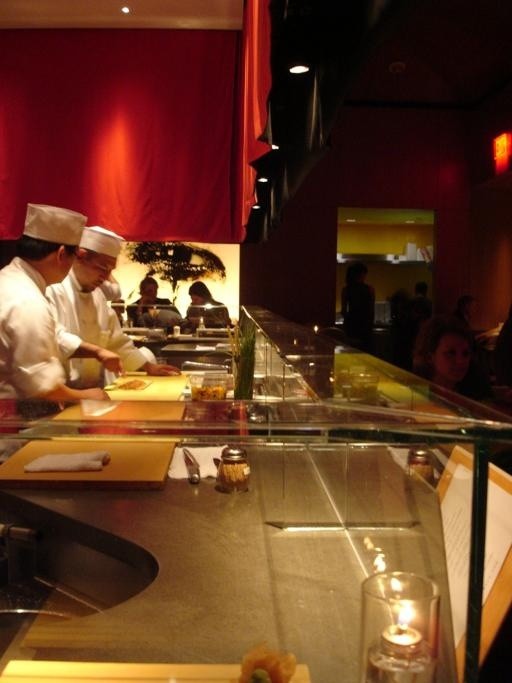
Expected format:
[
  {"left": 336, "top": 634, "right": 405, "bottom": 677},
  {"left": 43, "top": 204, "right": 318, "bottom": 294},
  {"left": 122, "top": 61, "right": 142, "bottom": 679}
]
[{"left": 360, "top": 571, "right": 442, "bottom": 682}]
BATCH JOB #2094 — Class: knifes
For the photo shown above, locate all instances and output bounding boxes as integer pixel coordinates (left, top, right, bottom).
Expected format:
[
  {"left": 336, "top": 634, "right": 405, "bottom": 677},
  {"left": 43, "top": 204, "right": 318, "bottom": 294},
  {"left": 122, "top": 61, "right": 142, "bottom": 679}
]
[{"left": 182, "top": 448, "right": 201, "bottom": 483}]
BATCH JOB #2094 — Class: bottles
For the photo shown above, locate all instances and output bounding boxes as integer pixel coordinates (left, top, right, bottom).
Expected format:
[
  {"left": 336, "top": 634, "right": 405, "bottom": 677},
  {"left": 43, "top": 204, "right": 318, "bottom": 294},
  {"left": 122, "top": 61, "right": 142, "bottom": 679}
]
[
  {"left": 405, "top": 445, "right": 435, "bottom": 484},
  {"left": 217, "top": 445, "right": 250, "bottom": 494}
]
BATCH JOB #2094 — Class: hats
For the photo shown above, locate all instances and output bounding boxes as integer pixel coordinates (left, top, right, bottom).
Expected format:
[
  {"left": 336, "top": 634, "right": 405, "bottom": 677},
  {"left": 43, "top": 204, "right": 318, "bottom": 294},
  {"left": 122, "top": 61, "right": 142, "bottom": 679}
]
[
  {"left": 22, "top": 202, "right": 88, "bottom": 246},
  {"left": 78, "top": 225, "right": 126, "bottom": 258}
]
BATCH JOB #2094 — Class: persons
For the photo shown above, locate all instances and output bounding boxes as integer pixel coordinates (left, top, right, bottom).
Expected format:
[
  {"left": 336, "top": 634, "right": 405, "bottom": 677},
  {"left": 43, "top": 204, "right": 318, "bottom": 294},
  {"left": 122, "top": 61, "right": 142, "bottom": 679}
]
[
  {"left": 0, "top": 201, "right": 112, "bottom": 432},
  {"left": 340, "top": 259, "right": 375, "bottom": 355},
  {"left": 126, "top": 275, "right": 182, "bottom": 332},
  {"left": 41, "top": 225, "right": 183, "bottom": 381},
  {"left": 386, "top": 281, "right": 512, "bottom": 476},
  {"left": 183, "top": 280, "right": 231, "bottom": 334}
]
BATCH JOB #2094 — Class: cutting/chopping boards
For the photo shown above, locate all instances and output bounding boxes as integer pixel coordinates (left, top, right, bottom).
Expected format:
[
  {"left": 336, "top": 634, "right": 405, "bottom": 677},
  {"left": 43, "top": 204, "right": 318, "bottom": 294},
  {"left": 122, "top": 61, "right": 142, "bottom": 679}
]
[
  {"left": 53, "top": 399, "right": 187, "bottom": 423},
  {"left": 101, "top": 366, "right": 188, "bottom": 400},
  {"left": 0, "top": 434, "right": 176, "bottom": 490}
]
